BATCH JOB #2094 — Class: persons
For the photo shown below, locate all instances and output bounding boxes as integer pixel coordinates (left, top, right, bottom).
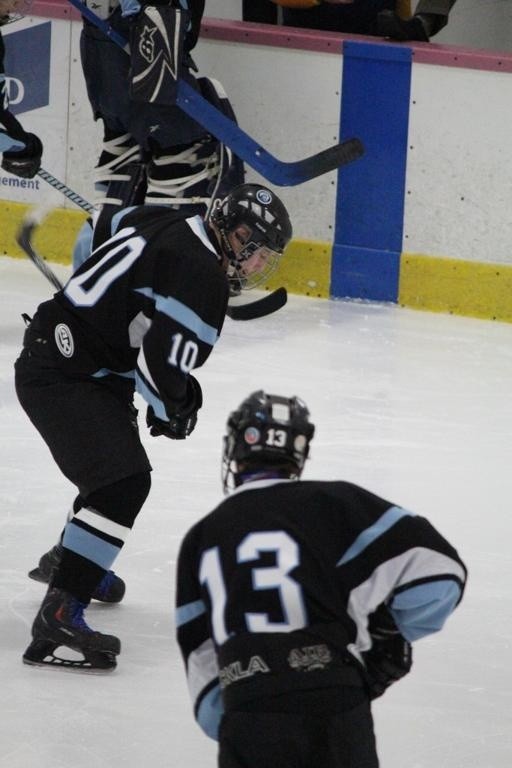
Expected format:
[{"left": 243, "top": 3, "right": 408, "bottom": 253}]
[
  {"left": 241, "top": 1, "right": 457, "bottom": 43},
  {"left": 175, "top": 390, "right": 467, "bottom": 767},
  {"left": 75, "top": 0, "right": 245, "bottom": 269},
  {"left": 0, "top": 35, "right": 42, "bottom": 179},
  {"left": 14, "top": 183, "right": 292, "bottom": 673}
]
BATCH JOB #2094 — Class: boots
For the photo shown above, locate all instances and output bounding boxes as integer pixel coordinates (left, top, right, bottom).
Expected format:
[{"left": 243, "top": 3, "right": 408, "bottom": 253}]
[
  {"left": 40, "top": 543, "right": 126, "bottom": 603},
  {"left": 31, "top": 585, "right": 120, "bottom": 655}
]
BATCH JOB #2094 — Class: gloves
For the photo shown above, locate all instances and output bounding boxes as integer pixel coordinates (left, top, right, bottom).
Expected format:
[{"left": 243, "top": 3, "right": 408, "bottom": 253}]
[
  {"left": 6, "top": 129, "right": 43, "bottom": 179},
  {"left": 361, "top": 614, "right": 412, "bottom": 683},
  {"left": 147, "top": 374, "right": 202, "bottom": 439}
]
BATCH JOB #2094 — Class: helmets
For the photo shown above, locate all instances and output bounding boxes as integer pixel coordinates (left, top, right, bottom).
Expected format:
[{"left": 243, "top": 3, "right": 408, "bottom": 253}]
[
  {"left": 210, "top": 184, "right": 293, "bottom": 290},
  {"left": 220, "top": 391, "right": 314, "bottom": 495}
]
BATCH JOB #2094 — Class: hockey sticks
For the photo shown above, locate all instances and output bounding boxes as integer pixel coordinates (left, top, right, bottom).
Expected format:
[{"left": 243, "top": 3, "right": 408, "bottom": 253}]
[
  {"left": 37, "top": 168, "right": 288, "bottom": 320},
  {"left": 66, "top": 0, "right": 363, "bottom": 187},
  {"left": 15, "top": 191, "right": 72, "bottom": 294}
]
